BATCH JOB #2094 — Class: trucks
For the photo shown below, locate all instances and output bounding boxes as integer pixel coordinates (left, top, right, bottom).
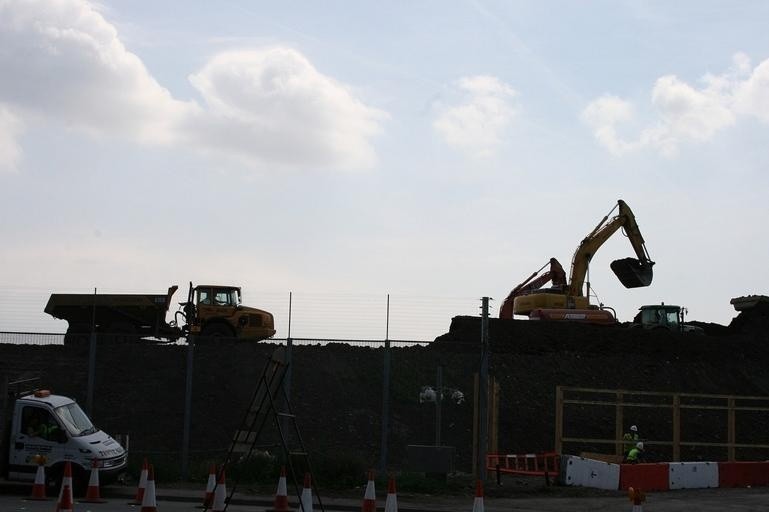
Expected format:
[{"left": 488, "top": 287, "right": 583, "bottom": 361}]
[
  {"left": 43, "top": 281, "right": 276, "bottom": 346},
  {"left": 1, "top": 376, "right": 127, "bottom": 494}
]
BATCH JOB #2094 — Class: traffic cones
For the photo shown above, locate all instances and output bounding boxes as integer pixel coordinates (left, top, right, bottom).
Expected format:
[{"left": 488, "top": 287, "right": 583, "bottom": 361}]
[
  {"left": 128, "top": 457, "right": 159, "bottom": 512},
  {"left": 362, "top": 468, "right": 398, "bottom": 512},
  {"left": 196, "top": 463, "right": 229, "bottom": 512},
  {"left": 472, "top": 479, "right": 484, "bottom": 512},
  {"left": 267, "top": 465, "right": 313, "bottom": 512},
  {"left": 21, "top": 454, "right": 108, "bottom": 512}
]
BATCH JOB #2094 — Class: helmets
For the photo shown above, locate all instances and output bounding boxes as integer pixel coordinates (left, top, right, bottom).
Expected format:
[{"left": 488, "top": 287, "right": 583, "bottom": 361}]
[
  {"left": 636, "top": 442, "right": 643, "bottom": 450},
  {"left": 630, "top": 425, "right": 638, "bottom": 431}
]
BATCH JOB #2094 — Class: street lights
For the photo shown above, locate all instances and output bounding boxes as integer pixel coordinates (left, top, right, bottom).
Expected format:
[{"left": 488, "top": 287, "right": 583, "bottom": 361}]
[{"left": 418, "top": 365, "right": 465, "bottom": 482}]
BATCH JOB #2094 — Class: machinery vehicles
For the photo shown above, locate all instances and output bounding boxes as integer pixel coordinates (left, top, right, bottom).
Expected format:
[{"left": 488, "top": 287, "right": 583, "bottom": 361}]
[{"left": 500, "top": 199, "right": 702, "bottom": 333}]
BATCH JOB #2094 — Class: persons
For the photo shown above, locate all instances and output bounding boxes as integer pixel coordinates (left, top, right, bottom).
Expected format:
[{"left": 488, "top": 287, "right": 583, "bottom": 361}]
[
  {"left": 622, "top": 441, "right": 645, "bottom": 462},
  {"left": 27, "top": 415, "right": 42, "bottom": 437},
  {"left": 623, "top": 425, "right": 641, "bottom": 454}
]
